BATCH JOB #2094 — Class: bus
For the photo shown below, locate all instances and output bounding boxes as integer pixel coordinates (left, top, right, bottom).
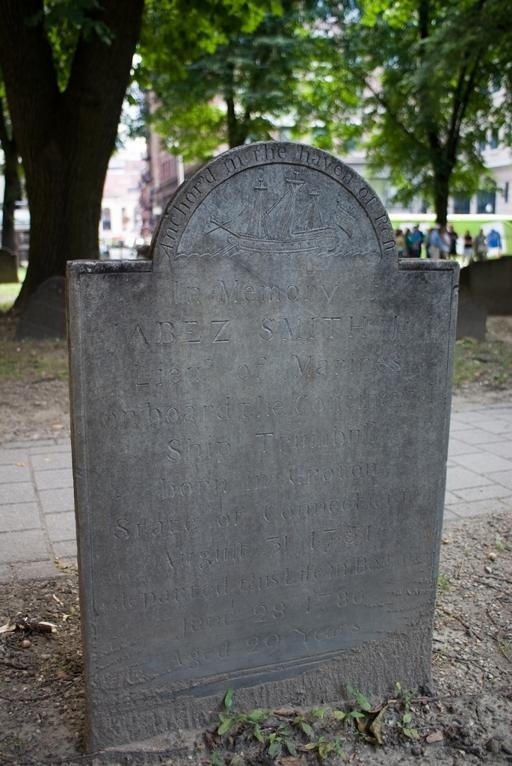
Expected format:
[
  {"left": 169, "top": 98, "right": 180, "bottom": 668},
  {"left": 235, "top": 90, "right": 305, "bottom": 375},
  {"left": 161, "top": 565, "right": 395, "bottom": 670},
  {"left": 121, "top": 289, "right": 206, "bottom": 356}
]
[{"left": 386, "top": 214, "right": 512, "bottom": 269}]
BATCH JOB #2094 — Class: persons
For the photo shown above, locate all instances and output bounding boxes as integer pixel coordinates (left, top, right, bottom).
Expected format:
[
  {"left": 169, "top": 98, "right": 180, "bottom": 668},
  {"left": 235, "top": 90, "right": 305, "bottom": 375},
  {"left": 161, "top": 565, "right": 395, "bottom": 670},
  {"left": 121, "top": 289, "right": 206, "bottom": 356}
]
[
  {"left": 133, "top": 226, "right": 154, "bottom": 259},
  {"left": 391, "top": 220, "right": 502, "bottom": 263}
]
